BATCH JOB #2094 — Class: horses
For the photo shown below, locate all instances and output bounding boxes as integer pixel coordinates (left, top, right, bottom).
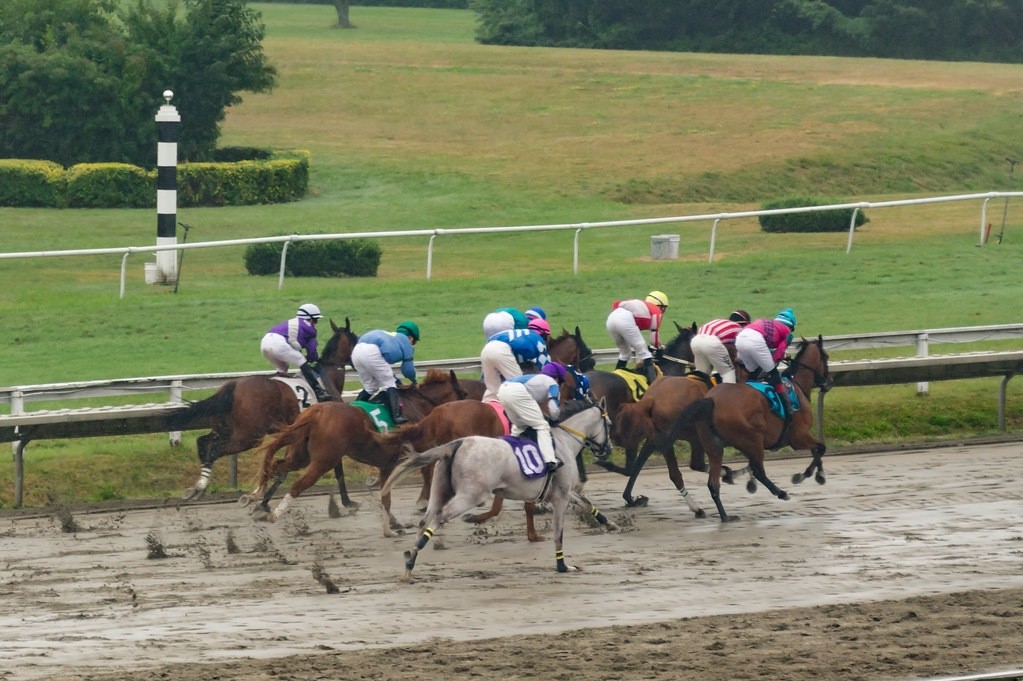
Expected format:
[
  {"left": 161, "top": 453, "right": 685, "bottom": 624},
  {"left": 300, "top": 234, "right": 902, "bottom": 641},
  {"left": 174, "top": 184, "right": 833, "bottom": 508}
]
[
  {"left": 456, "top": 327, "right": 596, "bottom": 495},
  {"left": 612, "top": 365, "right": 758, "bottom": 518},
  {"left": 160, "top": 316, "right": 361, "bottom": 510},
  {"left": 584, "top": 321, "right": 697, "bottom": 478},
  {"left": 377, "top": 397, "right": 620, "bottom": 582},
  {"left": 671, "top": 334, "right": 835, "bottom": 524},
  {"left": 366, "top": 359, "right": 579, "bottom": 542},
  {"left": 238, "top": 368, "right": 469, "bottom": 523}
]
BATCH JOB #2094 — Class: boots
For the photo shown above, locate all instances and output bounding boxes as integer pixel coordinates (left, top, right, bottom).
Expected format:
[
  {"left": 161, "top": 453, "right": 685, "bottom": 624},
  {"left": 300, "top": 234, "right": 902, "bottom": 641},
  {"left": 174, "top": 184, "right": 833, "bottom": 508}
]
[
  {"left": 644, "top": 357, "right": 656, "bottom": 386},
  {"left": 748, "top": 366, "right": 762, "bottom": 382},
  {"left": 386, "top": 386, "right": 408, "bottom": 425},
  {"left": 767, "top": 366, "right": 795, "bottom": 419},
  {"left": 299, "top": 362, "right": 333, "bottom": 402},
  {"left": 615, "top": 359, "right": 628, "bottom": 370}
]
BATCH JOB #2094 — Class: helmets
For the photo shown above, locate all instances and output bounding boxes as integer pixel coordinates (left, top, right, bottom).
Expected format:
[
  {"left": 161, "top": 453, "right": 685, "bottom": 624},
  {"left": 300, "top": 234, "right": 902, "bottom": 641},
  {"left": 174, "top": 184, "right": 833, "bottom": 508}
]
[
  {"left": 542, "top": 360, "right": 566, "bottom": 383},
  {"left": 296, "top": 304, "right": 324, "bottom": 319},
  {"left": 396, "top": 321, "right": 420, "bottom": 343},
  {"left": 525, "top": 306, "right": 546, "bottom": 320},
  {"left": 528, "top": 319, "right": 551, "bottom": 336},
  {"left": 646, "top": 291, "right": 669, "bottom": 307},
  {"left": 775, "top": 310, "right": 796, "bottom": 332},
  {"left": 733, "top": 310, "right": 751, "bottom": 326}
]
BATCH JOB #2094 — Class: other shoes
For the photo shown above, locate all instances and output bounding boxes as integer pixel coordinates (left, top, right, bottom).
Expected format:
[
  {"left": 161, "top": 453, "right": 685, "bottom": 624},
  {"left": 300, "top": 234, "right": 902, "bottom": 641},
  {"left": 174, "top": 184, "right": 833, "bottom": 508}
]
[{"left": 546, "top": 458, "right": 562, "bottom": 472}]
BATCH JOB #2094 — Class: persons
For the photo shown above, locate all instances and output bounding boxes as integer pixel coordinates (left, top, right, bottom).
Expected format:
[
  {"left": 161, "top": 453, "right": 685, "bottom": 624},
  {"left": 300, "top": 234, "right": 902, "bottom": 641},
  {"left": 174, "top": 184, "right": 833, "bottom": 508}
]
[
  {"left": 735, "top": 307, "right": 797, "bottom": 415},
  {"left": 690, "top": 309, "right": 751, "bottom": 383},
  {"left": 480, "top": 318, "right": 551, "bottom": 402},
  {"left": 497, "top": 361, "right": 567, "bottom": 469},
  {"left": 606, "top": 291, "right": 668, "bottom": 386},
  {"left": 481, "top": 306, "right": 546, "bottom": 381},
  {"left": 261, "top": 303, "right": 334, "bottom": 403},
  {"left": 351, "top": 320, "right": 419, "bottom": 424}
]
